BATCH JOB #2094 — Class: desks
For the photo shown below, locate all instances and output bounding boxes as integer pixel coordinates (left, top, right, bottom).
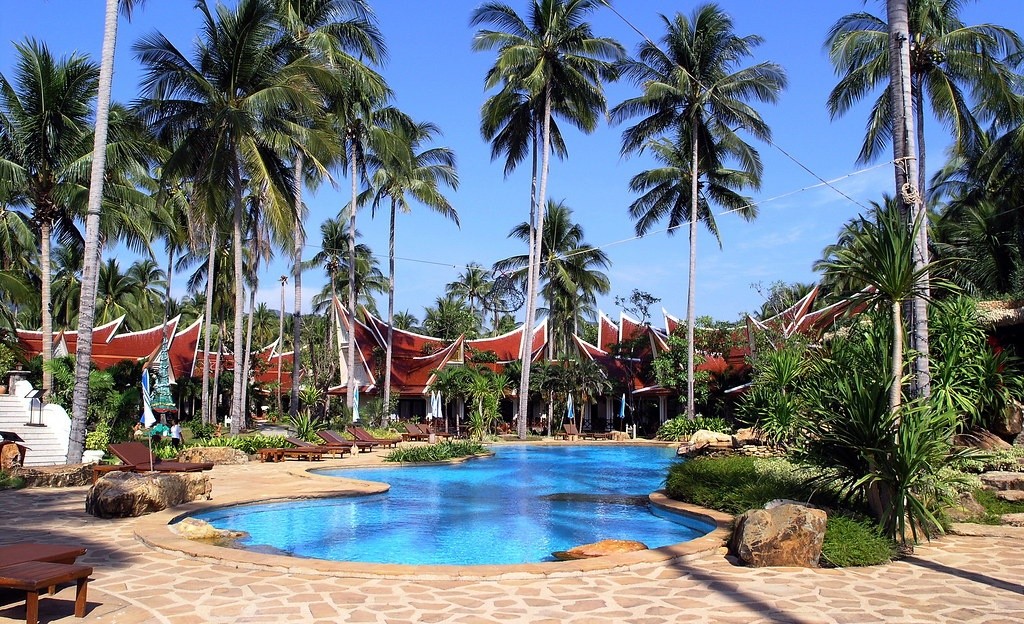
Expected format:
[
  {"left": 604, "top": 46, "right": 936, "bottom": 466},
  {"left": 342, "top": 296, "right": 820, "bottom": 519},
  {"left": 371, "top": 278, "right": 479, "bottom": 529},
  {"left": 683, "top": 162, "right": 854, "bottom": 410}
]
[
  {"left": 93, "top": 465, "right": 135, "bottom": 486},
  {"left": 0, "top": 560, "right": 93, "bottom": 624},
  {"left": 402, "top": 433, "right": 419, "bottom": 441},
  {"left": 257, "top": 448, "right": 285, "bottom": 463},
  {"left": 606, "top": 433, "right": 615, "bottom": 440}
]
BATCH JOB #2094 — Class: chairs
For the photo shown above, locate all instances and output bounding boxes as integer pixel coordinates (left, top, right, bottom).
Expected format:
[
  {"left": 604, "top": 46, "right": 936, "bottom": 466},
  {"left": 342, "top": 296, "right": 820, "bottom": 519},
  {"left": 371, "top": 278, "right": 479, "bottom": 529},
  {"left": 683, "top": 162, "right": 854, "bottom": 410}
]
[
  {"left": 285, "top": 424, "right": 606, "bottom": 463},
  {"left": 107, "top": 443, "right": 218, "bottom": 472},
  {"left": 0, "top": 546, "right": 87, "bottom": 587}
]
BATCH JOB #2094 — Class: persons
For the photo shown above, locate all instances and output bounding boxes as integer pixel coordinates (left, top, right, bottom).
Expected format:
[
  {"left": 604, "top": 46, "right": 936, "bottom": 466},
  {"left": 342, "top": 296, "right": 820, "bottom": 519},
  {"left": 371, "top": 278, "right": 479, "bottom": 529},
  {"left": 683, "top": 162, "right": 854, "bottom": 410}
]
[{"left": 171, "top": 419, "right": 184, "bottom": 450}]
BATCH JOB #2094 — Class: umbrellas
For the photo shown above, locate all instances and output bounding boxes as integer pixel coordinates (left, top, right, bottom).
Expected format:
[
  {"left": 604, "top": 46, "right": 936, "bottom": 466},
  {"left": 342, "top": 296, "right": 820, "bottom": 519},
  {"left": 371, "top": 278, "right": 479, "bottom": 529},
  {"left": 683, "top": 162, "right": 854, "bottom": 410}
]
[
  {"left": 140, "top": 369, "right": 156, "bottom": 472},
  {"left": 353, "top": 381, "right": 360, "bottom": 447},
  {"left": 567, "top": 393, "right": 574, "bottom": 434},
  {"left": 431, "top": 390, "right": 443, "bottom": 433},
  {"left": 619, "top": 393, "right": 625, "bottom": 434}
]
